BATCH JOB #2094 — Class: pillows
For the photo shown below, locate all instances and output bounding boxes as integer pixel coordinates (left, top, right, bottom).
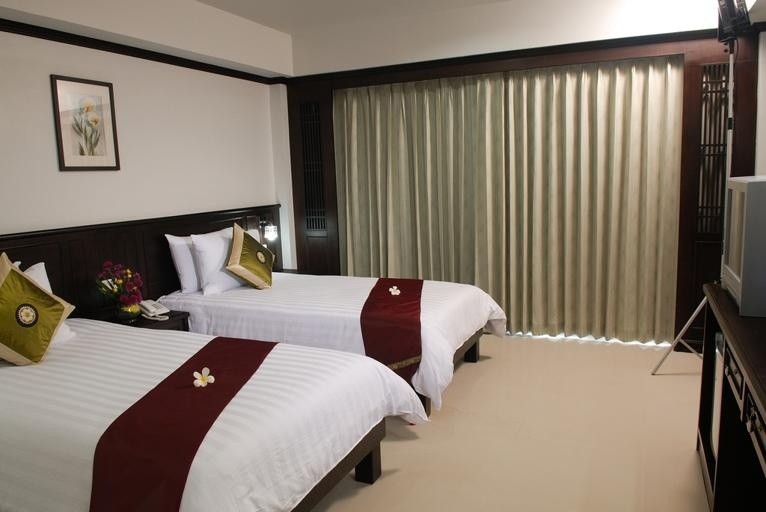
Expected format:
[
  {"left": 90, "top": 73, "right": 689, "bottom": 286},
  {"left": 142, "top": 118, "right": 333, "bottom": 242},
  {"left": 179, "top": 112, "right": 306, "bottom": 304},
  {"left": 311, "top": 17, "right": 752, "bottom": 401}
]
[
  {"left": 164, "top": 222, "right": 277, "bottom": 297},
  {"left": 0, "top": 251, "right": 78, "bottom": 366}
]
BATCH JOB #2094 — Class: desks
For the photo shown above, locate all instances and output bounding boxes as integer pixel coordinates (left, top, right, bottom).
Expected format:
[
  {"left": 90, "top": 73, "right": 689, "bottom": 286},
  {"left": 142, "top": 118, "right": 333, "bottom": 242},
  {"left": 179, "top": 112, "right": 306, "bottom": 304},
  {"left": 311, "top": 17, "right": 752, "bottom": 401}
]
[{"left": 696, "top": 282, "right": 766, "bottom": 511}]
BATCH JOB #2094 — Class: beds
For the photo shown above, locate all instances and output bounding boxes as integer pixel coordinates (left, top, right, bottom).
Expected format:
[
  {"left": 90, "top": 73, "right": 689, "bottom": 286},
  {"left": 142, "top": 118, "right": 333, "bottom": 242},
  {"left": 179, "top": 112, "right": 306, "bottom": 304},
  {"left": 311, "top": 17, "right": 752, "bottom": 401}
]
[
  {"left": 158, "top": 272, "right": 485, "bottom": 421},
  {"left": 0, "top": 319, "right": 386, "bottom": 512}
]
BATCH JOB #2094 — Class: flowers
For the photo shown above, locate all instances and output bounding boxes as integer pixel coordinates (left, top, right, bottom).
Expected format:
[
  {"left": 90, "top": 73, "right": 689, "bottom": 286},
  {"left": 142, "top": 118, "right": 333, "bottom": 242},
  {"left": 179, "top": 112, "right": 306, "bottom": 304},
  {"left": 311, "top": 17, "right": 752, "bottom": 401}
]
[
  {"left": 96, "top": 260, "right": 144, "bottom": 305},
  {"left": 389, "top": 286, "right": 401, "bottom": 296},
  {"left": 193, "top": 367, "right": 215, "bottom": 388}
]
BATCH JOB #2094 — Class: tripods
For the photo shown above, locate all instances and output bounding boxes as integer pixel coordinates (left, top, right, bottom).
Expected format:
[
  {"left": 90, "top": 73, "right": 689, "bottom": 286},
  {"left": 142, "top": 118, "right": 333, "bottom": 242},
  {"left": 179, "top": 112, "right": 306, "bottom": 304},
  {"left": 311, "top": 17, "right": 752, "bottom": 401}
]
[{"left": 651, "top": 52, "right": 736, "bottom": 377}]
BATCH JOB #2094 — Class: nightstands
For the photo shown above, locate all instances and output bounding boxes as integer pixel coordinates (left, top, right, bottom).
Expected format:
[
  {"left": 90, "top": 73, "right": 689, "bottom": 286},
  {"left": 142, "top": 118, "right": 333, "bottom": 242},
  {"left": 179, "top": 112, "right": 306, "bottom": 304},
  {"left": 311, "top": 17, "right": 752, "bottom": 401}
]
[{"left": 109, "top": 311, "right": 190, "bottom": 330}]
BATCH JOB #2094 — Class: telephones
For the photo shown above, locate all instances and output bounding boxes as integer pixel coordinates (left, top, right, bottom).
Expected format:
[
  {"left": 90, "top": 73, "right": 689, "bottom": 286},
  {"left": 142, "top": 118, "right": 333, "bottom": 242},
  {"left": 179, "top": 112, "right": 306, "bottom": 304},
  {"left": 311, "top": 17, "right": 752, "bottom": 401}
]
[{"left": 140, "top": 300, "right": 170, "bottom": 317}]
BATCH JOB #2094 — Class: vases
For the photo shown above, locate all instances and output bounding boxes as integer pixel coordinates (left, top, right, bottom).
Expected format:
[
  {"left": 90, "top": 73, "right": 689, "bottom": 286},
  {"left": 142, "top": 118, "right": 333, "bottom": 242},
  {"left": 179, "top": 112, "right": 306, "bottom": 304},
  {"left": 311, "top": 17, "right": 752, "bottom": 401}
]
[{"left": 114, "top": 304, "right": 142, "bottom": 318}]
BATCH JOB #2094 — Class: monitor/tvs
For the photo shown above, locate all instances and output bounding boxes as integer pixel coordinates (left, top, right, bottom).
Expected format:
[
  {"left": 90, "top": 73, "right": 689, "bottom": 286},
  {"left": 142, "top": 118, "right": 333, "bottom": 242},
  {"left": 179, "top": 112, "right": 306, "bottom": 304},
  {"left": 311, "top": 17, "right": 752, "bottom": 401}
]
[{"left": 720, "top": 175, "right": 766, "bottom": 318}]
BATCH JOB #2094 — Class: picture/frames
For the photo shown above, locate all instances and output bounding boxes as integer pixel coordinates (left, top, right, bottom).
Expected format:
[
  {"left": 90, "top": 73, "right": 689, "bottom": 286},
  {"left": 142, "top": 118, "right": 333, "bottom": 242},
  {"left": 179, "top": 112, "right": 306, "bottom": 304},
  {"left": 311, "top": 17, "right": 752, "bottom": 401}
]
[{"left": 51, "top": 75, "right": 120, "bottom": 170}]
